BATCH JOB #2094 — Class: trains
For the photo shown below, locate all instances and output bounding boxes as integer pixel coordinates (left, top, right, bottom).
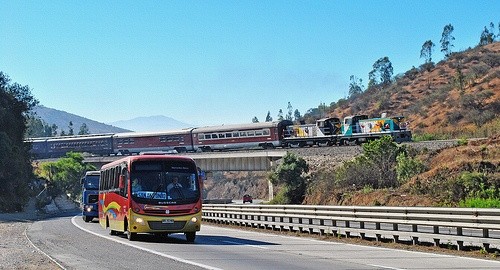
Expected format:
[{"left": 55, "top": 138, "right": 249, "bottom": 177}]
[{"left": 24, "top": 110, "right": 411, "bottom": 159}]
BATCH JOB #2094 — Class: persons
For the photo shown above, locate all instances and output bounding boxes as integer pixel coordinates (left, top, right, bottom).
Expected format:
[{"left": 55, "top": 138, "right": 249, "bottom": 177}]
[
  {"left": 132, "top": 177, "right": 142, "bottom": 192},
  {"left": 167, "top": 176, "right": 183, "bottom": 192}
]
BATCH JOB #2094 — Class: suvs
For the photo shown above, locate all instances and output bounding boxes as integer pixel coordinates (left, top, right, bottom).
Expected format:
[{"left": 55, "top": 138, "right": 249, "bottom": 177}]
[{"left": 242, "top": 194, "right": 253, "bottom": 204}]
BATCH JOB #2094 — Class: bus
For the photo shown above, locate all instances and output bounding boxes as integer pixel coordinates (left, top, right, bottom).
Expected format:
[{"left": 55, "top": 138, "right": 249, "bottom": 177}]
[
  {"left": 99, "top": 156, "right": 202, "bottom": 243},
  {"left": 81, "top": 170, "right": 102, "bottom": 223}
]
